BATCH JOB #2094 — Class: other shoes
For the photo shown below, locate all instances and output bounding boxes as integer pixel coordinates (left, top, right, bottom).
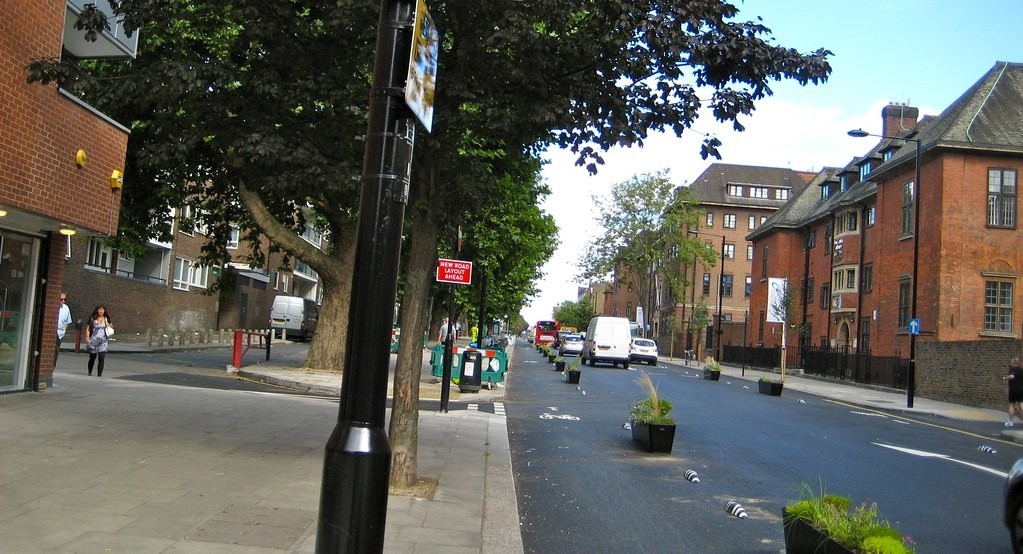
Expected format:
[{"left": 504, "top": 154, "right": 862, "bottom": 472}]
[{"left": 1005, "top": 422, "right": 1013, "bottom": 426}]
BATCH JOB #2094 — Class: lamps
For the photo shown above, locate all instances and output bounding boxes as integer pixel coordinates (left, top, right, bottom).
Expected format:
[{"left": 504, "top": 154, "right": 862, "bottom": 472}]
[{"left": 59, "top": 226, "right": 77, "bottom": 236}]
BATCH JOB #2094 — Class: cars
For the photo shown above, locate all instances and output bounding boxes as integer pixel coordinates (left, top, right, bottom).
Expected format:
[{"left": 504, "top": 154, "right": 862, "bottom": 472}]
[{"left": 1002, "top": 457, "right": 1023, "bottom": 554}]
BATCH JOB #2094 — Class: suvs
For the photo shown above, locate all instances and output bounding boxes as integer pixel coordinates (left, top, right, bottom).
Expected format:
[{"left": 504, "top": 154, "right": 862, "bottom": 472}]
[
  {"left": 631, "top": 336, "right": 658, "bottom": 367},
  {"left": 559, "top": 331, "right": 584, "bottom": 356}
]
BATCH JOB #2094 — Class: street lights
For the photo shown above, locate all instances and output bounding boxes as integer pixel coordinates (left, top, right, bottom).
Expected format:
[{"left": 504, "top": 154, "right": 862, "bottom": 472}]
[
  {"left": 689, "top": 229, "right": 726, "bottom": 361},
  {"left": 847, "top": 128, "right": 922, "bottom": 408}
]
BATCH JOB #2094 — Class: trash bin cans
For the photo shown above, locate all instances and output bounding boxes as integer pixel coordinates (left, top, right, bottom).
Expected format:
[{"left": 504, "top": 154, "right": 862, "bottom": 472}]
[{"left": 459, "top": 351, "right": 482, "bottom": 393}]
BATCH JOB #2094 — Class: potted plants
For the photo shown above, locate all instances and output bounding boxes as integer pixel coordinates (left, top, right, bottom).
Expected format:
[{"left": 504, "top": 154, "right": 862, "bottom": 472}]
[
  {"left": 534, "top": 343, "right": 582, "bottom": 384},
  {"left": 630, "top": 371, "right": 677, "bottom": 455},
  {"left": 780, "top": 474, "right": 917, "bottom": 554},
  {"left": 758, "top": 377, "right": 785, "bottom": 396},
  {"left": 703, "top": 356, "right": 721, "bottom": 381}
]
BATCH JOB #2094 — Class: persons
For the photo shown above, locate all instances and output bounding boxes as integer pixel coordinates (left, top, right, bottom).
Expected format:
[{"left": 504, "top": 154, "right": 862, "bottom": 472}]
[
  {"left": 1002, "top": 358, "right": 1023, "bottom": 426},
  {"left": 468, "top": 321, "right": 493, "bottom": 343},
  {"left": 85, "top": 304, "right": 114, "bottom": 377},
  {"left": 437, "top": 317, "right": 456, "bottom": 345},
  {"left": 453, "top": 321, "right": 461, "bottom": 341},
  {"left": 54, "top": 292, "right": 72, "bottom": 368}
]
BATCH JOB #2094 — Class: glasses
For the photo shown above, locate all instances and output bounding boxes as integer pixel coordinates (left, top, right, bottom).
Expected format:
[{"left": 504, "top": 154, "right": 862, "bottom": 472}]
[{"left": 60, "top": 299, "right": 66, "bottom": 301}]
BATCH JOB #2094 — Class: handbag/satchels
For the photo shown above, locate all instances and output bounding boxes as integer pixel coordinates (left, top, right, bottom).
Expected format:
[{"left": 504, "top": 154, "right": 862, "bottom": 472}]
[{"left": 105, "top": 317, "right": 114, "bottom": 338}]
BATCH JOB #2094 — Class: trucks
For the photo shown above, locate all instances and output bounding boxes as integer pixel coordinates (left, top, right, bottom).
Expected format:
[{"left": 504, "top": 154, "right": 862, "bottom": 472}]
[{"left": 561, "top": 325, "right": 577, "bottom": 332}]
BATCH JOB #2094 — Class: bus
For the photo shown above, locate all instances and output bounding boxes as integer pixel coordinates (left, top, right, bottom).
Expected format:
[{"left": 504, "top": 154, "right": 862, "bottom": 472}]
[{"left": 535, "top": 321, "right": 559, "bottom": 348}]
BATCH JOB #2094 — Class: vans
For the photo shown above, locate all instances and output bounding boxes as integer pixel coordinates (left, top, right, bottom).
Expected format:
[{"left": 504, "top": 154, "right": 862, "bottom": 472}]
[
  {"left": 269, "top": 295, "right": 319, "bottom": 342},
  {"left": 581, "top": 317, "right": 631, "bottom": 369}
]
